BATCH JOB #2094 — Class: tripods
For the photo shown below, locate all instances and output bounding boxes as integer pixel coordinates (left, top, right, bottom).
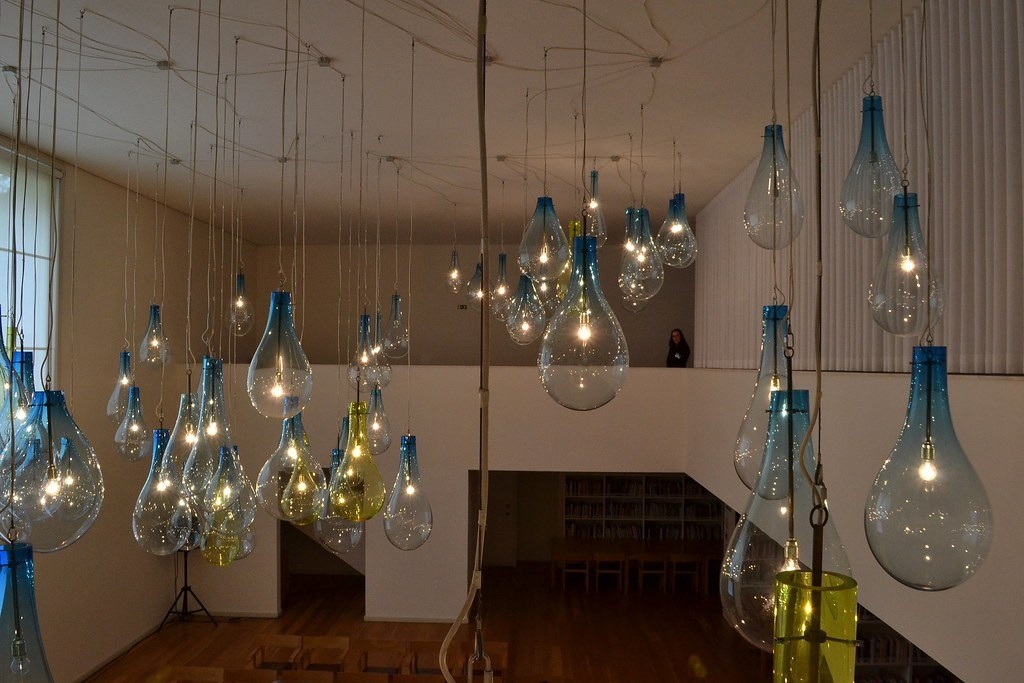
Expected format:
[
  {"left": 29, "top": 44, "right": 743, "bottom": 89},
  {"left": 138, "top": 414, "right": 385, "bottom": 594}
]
[{"left": 158, "top": 551, "right": 218, "bottom": 632}]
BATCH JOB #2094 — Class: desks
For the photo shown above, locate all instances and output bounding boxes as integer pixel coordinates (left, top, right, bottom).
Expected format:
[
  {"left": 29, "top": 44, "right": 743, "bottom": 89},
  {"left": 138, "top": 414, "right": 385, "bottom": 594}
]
[{"left": 553, "top": 537, "right": 708, "bottom": 591}]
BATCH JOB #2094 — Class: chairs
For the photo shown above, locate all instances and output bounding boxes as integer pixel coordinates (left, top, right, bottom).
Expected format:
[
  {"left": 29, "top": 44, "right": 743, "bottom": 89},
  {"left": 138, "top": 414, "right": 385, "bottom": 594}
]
[
  {"left": 561, "top": 552, "right": 702, "bottom": 595},
  {"left": 245, "top": 632, "right": 508, "bottom": 682}
]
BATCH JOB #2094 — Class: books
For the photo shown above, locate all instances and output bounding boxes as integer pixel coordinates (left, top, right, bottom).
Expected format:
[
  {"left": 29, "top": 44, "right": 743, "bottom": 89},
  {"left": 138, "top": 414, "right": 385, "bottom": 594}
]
[
  {"left": 565, "top": 476, "right": 724, "bottom": 541},
  {"left": 741, "top": 531, "right": 956, "bottom": 683}
]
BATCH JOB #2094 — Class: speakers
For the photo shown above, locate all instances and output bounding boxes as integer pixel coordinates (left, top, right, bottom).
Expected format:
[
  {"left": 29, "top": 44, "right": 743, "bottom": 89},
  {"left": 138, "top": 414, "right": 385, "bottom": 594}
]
[{"left": 174, "top": 516, "right": 199, "bottom": 546}]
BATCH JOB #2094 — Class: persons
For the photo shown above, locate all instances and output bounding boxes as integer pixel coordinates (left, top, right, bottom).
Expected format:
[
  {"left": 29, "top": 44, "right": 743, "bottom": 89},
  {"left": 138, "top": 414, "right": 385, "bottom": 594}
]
[{"left": 666, "top": 328, "right": 691, "bottom": 368}]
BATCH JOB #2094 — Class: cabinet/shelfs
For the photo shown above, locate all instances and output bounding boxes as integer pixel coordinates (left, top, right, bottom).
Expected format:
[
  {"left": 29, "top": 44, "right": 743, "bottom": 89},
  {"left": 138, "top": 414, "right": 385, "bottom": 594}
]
[
  {"left": 563, "top": 473, "right": 724, "bottom": 542},
  {"left": 854, "top": 600, "right": 964, "bottom": 683}
]
